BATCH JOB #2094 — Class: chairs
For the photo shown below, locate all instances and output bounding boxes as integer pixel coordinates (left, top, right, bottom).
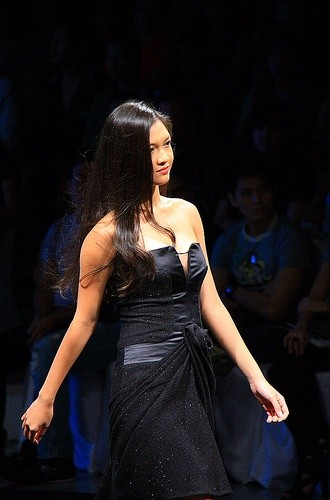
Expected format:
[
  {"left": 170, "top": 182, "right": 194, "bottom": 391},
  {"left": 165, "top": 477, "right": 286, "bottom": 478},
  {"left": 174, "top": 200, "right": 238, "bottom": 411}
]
[{"left": 14, "top": 329, "right": 329, "bottom": 494}]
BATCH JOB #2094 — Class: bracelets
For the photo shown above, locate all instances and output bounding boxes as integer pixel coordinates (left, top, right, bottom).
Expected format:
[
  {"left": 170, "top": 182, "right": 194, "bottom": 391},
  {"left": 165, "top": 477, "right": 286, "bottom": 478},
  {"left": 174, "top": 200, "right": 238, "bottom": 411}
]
[{"left": 225, "top": 281, "right": 239, "bottom": 300}]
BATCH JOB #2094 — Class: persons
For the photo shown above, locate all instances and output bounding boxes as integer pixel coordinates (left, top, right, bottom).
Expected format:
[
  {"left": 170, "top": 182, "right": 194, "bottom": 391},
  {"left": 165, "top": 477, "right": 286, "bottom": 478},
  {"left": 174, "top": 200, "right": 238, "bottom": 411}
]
[
  {"left": 208, "top": 167, "right": 308, "bottom": 490},
  {"left": 13, "top": 164, "right": 117, "bottom": 496},
  {"left": 20, "top": 101, "right": 290, "bottom": 500},
  {"left": 282, "top": 195, "right": 330, "bottom": 447}
]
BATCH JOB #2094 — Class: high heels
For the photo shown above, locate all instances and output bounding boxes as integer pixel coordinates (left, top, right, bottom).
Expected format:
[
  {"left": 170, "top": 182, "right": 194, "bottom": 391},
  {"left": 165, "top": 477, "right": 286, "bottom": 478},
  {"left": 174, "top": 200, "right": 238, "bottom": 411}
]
[
  {"left": 281, "top": 449, "right": 323, "bottom": 500},
  {"left": 315, "top": 436, "right": 330, "bottom": 500}
]
